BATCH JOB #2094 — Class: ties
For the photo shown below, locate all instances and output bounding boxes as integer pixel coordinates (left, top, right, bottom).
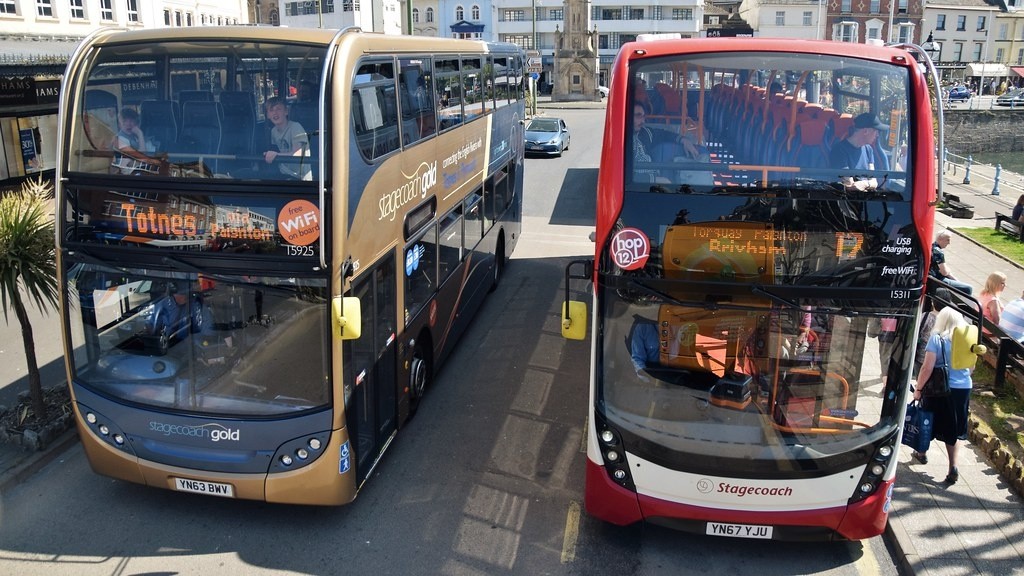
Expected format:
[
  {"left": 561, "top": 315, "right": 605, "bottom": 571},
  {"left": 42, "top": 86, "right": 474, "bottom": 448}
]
[{"left": 169, "top": 306, "right": 182, "bottom": 345}]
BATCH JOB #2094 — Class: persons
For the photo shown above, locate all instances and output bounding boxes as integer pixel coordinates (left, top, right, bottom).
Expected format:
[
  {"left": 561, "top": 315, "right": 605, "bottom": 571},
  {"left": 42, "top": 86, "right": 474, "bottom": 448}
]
[
  {"left": 974, "top": 269, "right": 1008, "bottom": 349},
  {"left": 631, "top": 321, "right": 696, "bottom": 387},
  {"left": 763, "top": 82, "right": 783, "bottom": 101},
  {"left": 113, "top": 107, "right": 170, "bottom": 176},
  {"left": 288, "top": 80, "right": 317, "bottom": 120},
  {"left": 997, "top": 289, "right": 1024, "bottom": 359},
  {"left": 990, "top": 79, "right": 1019, "bottom": 96},
  {"left": 767, "top": 306, "right": 812, "bottom": 389},
  {"left": 828, "top": 112, "right": 890, "bottom": 193},
  {"left": 877, "top": 287, "right": 976, "bottom": 484},
  {"left": 262, "top": 96, "right": 313, "bottom": 182},
  {"left": 633, "top": 100, "right": 700, "bottom": 194},
  {"left": 931, "top": 230, "right": 973, "bottom": 298},
  {"left": 149, "top": 279, "right": 216, "bottom": 360},
  {"left": 1011, "top": 194, "right": 1024, "bottom": 223}
]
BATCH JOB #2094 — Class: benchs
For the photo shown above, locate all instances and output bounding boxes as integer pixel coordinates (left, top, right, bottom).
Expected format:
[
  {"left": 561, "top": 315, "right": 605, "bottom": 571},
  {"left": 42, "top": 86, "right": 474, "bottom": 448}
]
[
  {"left": 936, "top": 188, "right": 975, "bottom": 216},
  {"left": 994, "top": 211, "right": 1024, "bottom": 241}
]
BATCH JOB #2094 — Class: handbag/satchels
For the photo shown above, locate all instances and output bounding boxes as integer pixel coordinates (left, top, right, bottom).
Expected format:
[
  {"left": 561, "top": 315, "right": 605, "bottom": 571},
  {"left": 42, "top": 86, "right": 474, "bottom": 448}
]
[
  {"left": 901, "top": 398, "right": 934, "bottom": 452},
  {"left": 916, "top": 333, "right": 951, "bottom": 398},
  {"left": 868, "top": 319, "right": 882, "bottom": 338}
]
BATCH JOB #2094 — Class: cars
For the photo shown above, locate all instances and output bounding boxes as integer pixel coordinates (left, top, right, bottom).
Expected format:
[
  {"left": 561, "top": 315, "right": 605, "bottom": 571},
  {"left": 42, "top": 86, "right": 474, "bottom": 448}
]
[
  {"left": 524, "top": 117, "right": 570, "bottom": 157},
  {"left": 599, "top": 85, "right": 609, "bottom": 98},
  {"left": 116, "top": 278, "right": 204, "bottom": 355},
  {"left": 945, "top": 86, "right": 970, "bottom": 103},
  {"left": 997, "top": 89, "right": 1024, "bottom": 106}
]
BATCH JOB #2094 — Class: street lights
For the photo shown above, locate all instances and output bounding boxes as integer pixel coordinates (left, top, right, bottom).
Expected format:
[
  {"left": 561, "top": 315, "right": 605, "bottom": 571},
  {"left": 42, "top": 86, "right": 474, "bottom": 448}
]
[{"left": 919, "top": 30, "right": 940, "bottom": 83}]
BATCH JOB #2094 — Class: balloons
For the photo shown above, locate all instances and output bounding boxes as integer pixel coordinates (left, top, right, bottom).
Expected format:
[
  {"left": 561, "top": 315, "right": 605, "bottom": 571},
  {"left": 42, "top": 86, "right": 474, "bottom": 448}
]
[{"left": 289, "top": 86, "right": 298, "bottom": 95}]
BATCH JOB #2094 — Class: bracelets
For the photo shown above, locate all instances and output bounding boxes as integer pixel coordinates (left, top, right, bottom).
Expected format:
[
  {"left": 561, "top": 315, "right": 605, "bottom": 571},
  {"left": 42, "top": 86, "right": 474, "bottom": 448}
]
[
  {"left": 916, "top": 389, "right": 922, "bottom": 392},
  {"left": 867, "top": 180, "right": 872, "bottom": 188},
  {"left": 655, "top": 378, "right": 660, "bottom": 386}
]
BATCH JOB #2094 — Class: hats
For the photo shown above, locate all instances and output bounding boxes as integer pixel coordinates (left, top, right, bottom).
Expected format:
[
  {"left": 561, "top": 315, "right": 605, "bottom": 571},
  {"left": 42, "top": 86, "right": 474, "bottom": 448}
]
[{"left": 854, "top": 112, "right": 890, "bottom": 131}]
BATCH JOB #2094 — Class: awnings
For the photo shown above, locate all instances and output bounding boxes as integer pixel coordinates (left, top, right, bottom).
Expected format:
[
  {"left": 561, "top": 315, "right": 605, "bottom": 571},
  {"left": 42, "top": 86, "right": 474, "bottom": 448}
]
[{"left": 1011, "top": 64, "right": 1024, "bottom": 79}]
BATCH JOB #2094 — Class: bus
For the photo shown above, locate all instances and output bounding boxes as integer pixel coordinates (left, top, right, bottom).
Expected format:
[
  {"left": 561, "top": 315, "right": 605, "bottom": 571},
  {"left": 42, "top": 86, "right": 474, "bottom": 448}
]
[
  {"left": 560, "top": 33, "right": 987, "bottom": 541},
  {"left": 55, "top": 26, "right": 525, "bottom": 504}
]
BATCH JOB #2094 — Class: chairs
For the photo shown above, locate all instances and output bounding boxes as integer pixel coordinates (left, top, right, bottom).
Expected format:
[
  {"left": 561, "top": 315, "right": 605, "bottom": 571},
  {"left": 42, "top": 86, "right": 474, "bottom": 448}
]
[
  {"left": 651, "top": 80, "right": 888, "bottom": 183},
  {"left": 138, "top": 83, "right": 442, "bottom": 167}
]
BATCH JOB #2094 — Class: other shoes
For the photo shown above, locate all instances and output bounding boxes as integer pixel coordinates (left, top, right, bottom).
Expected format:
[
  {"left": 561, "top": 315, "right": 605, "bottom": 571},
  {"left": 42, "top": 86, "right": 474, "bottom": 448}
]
[
  {"left": 945, "top": 467, "right": 959, "bottom": 482},
  {"left": 878, "top": 385, "right": 886, "bottom": 398},
  {"left": 913, "top": 450, "right": 927, "bottom": 464}
]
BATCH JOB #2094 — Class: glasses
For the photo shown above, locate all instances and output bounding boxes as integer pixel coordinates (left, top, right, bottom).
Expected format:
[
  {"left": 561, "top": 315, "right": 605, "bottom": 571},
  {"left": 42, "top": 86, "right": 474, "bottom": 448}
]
[{"left": 634, "top": 114, "right": 647, "bottom": 119}]
